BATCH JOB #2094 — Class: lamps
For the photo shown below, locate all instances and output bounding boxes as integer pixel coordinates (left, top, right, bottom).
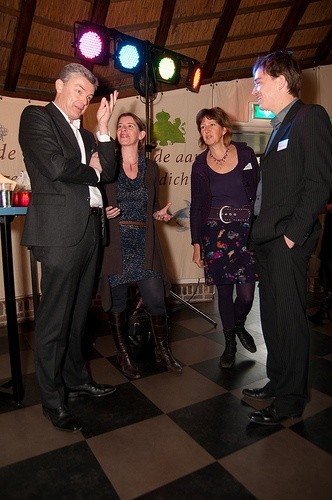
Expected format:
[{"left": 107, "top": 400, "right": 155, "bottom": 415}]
[{"left": 76, "top": 19, "right": 205, "bottom": 94}]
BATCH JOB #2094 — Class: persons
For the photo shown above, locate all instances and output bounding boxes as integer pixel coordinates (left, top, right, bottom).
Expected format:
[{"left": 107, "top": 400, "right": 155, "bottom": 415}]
[
  {"left": 316, "top": 198, "right": 332, "bottom": 323},
  {"left": 189, "top": 106, "right": 260, "bottom": 369},
  {"left": 17, "top": 63, "right": 119, "bottom": 434},
  {"left": 240, "top": 52, "right": 332, "bottom": 426},
  {"left": 98, "top": 111, "right": 185, "bottom": 381}
]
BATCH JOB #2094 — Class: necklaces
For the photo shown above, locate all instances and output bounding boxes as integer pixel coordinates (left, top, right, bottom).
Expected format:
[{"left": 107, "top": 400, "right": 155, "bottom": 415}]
[
  {"left": 208, "top": 146, "right": 230, "bottom": 167},
  {"left": 121, "top": 155, "right": 137, "bottom": 171}
]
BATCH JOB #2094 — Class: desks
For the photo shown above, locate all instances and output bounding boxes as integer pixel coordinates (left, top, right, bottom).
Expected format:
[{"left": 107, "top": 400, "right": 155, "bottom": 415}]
[{"left": 0, "top": 204, "right": 42, "bottom": 413}]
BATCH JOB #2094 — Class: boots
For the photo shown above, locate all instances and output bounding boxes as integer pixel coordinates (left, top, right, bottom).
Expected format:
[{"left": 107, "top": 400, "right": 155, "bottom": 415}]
[
  {"left": 148, "top": 314, "right": 182, "bottom": 373},
  {"left": 111, "top": 310, "right": 140, "bottom": 379}
]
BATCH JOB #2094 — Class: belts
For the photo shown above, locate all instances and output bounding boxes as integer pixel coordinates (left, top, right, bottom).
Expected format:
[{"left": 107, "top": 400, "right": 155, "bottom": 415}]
[{"left": 207, "top": 204, "right": 254, "bottom": 224}]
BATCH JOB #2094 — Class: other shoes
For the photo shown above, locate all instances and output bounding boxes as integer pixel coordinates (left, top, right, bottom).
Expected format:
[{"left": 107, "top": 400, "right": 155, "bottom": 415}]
[
  {"left": 236, "top": 325, "right": 257, "bottom": 353},
  {"left": 218, "top": 329, "right": 237, "bottom": 369}
]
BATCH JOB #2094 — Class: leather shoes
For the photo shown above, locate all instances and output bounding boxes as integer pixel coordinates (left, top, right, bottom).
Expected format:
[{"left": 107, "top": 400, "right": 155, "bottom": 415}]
[
  {"left": 242, "top": 387, "right": 278, "bottom": 400},
  {"left": 64, "top": 378, "right": 117, "bottom": 398},
  {"left": 248, "top": 397, "right": 304, "bottom": 426},
  {"left": 42, "top": 404, "right": 82, "bottom": 433}
]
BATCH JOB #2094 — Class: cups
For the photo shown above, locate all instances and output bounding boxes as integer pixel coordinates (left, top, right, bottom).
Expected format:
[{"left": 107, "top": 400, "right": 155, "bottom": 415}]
[{"left": 0, "top": 182, "right": 32, "bottom": 208}]
